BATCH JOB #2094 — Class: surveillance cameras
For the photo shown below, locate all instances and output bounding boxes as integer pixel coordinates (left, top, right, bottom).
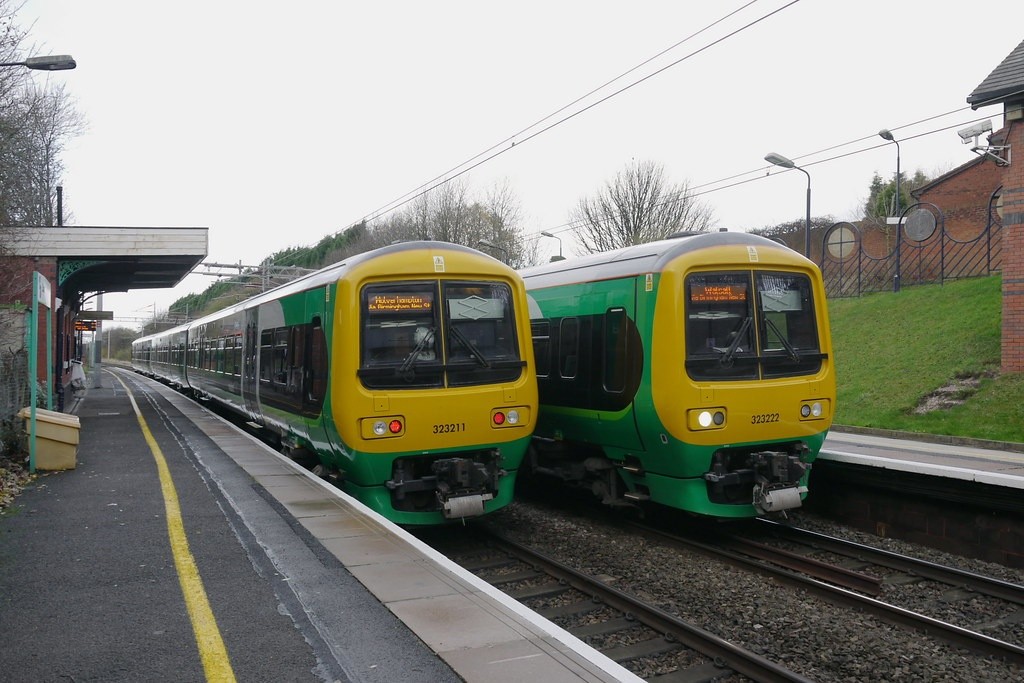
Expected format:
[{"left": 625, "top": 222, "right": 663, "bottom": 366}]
[{"left": 958, "top": 119, "right": 992, "bottom": 139}]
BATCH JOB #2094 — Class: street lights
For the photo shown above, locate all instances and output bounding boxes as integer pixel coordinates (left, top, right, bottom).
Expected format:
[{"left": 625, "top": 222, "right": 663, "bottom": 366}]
[
  {"left": 764, "top": 152, "right": 812, "bottom": 260},
  {"left": 541, "top": 231, "right": 562, "bottom": 257},
  {"left": 479, "top": 239, "right": 509, "bottom": 265},
  {"left": 878, "top": 128, "right": 902, "bottom": 294}
]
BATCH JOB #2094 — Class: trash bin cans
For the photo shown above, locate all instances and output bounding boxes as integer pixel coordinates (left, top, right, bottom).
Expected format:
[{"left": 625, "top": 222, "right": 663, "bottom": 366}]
[{"left": 24, "top": 407, "right": 80, "bottom": 471}]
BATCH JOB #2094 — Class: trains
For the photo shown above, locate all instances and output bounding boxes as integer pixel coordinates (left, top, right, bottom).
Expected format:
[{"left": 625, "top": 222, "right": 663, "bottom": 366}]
[
  {"left": 130, "top": 241, "right": 541, "bottom": 527},
  {"left": 510, "top": 229, "right": 837, "bottom": 520}
]
